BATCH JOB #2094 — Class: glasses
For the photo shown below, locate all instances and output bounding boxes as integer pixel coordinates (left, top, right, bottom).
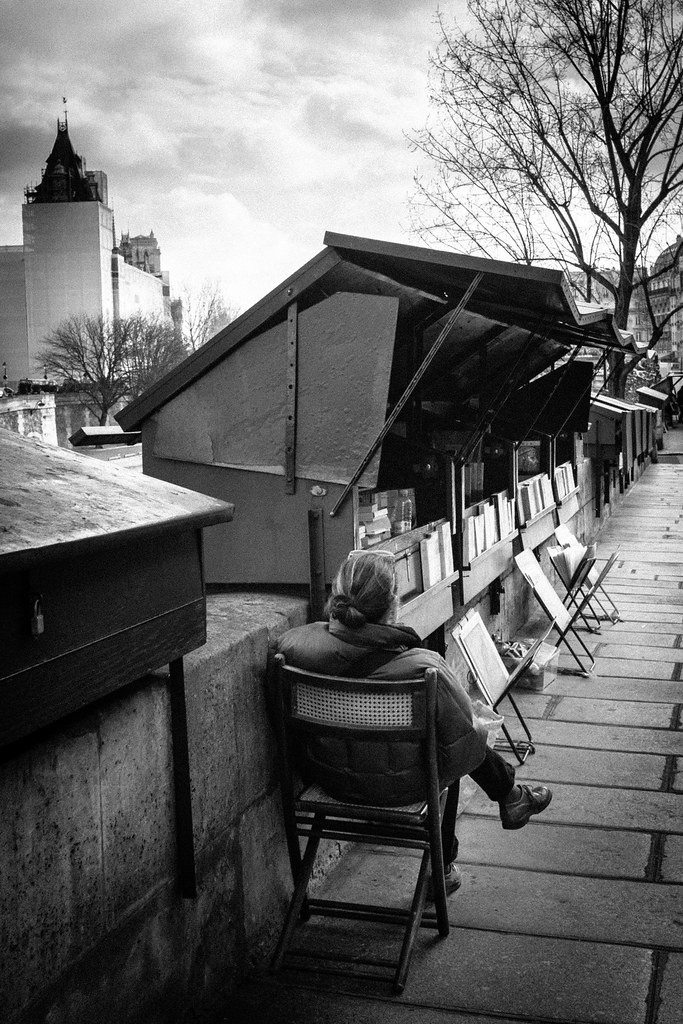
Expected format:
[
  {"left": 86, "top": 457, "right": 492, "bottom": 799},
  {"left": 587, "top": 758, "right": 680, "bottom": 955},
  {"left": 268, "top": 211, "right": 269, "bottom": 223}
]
[{"left": 348, "top": 550, "right": 396, "bottom": 573}]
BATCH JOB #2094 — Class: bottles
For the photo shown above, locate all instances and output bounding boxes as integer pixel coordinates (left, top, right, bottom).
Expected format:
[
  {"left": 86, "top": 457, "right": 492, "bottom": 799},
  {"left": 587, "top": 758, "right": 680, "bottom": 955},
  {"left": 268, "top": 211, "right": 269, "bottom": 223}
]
[{"left": 393, "top": 489, "right": 413, "bottom": 536}]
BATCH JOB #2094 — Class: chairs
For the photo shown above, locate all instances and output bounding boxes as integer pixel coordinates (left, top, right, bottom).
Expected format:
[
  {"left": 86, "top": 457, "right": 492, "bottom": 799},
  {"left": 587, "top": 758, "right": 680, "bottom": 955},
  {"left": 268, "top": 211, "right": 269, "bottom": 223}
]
[{"left": 269, "top": 652, "right": 459, "bottom": 995}]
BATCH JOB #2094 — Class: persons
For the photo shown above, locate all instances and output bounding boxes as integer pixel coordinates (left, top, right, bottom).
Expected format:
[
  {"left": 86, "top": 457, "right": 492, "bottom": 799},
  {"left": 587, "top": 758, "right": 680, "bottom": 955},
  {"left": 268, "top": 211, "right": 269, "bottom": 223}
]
[
  {"left": 270, "top": 549, "right": 554, "bottom": 902},
  {"left": 670, "top": 396, "right": 682, "bottom": 429}
]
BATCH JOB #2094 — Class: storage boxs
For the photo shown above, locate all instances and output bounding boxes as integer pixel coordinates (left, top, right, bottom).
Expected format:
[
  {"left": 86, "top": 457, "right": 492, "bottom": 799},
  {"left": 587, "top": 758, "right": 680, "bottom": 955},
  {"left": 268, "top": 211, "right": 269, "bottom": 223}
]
[
  {"left": 501, "top": 649, "right": 560, "bottom": 690},
  {"left": 359, "top": 488, "right": 416, "bottom": 548}
]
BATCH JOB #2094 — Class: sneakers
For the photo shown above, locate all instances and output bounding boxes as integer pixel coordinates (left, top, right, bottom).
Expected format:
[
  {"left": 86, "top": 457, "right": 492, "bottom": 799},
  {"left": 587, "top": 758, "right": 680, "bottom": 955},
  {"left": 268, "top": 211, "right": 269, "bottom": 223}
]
[
  {"left": 500, "top": 783, "right": 553, "bottom": 830},
  {"left": 430, "top": 863, "right": 461, "bottom": 899}
]
